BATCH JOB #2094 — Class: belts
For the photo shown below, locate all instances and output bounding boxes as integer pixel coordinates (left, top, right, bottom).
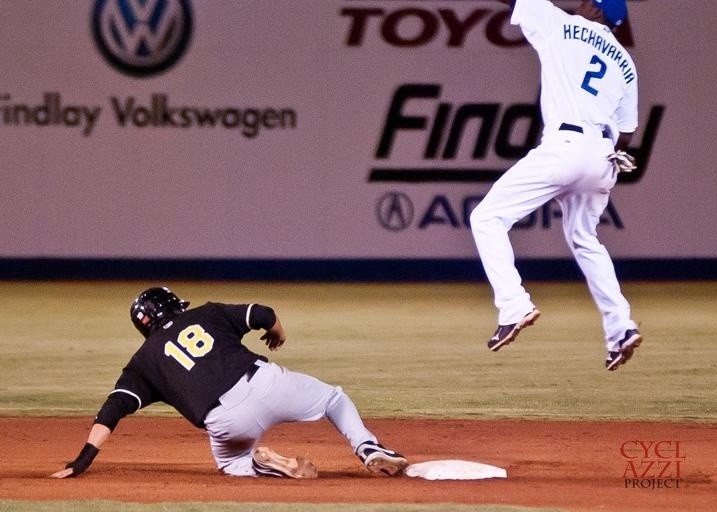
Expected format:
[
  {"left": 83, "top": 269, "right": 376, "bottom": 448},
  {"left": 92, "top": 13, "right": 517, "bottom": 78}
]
[
  {"left": 560, "top": 123, "right": 609, "bottom": 138},
  {"left": 219, "top": 355, "right": 269, "bottom": 403}
]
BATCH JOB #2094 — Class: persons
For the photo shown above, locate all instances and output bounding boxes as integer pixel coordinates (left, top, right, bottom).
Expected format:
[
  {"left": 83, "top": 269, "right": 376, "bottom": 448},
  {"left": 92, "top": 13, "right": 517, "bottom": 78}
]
[
  {"left": 48, "top": 285, "right": 407, "bottom": 482},
  {"left": 469, "top": 0, "right": 646, "bottom": 370}
]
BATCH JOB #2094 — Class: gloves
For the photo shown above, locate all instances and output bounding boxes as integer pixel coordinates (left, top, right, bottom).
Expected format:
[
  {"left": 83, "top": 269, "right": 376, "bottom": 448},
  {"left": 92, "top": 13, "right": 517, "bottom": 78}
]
[{"left": 608, "top": 148, "right": 639, "bottom": 173}]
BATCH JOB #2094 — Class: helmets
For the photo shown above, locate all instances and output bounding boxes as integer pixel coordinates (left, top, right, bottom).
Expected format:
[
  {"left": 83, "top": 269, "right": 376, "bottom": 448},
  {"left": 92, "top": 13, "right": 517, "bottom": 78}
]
[{"left": 131, "top": 285, "right": 191, "bottom": 336}]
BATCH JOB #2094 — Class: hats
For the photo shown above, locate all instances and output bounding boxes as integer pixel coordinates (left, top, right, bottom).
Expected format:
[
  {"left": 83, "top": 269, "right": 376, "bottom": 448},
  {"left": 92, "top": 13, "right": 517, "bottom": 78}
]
[{"left": 594, "top": 0, "right": 628, "bottom": 27}]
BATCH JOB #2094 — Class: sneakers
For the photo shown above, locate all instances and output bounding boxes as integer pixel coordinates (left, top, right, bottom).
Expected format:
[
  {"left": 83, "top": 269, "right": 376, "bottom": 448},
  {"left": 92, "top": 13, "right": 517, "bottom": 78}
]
[
  {"left": 487, "top": 307, "right": 541, "bottom": 352},
  {"left": 252, "top": 447, "right": 319, "bottom": 480},
  {"left": 606, "top": 329, "right": 644, "bottom": 372},
  {"left": 356, "top": 439, "right": 410, "bottom": 477}
]
